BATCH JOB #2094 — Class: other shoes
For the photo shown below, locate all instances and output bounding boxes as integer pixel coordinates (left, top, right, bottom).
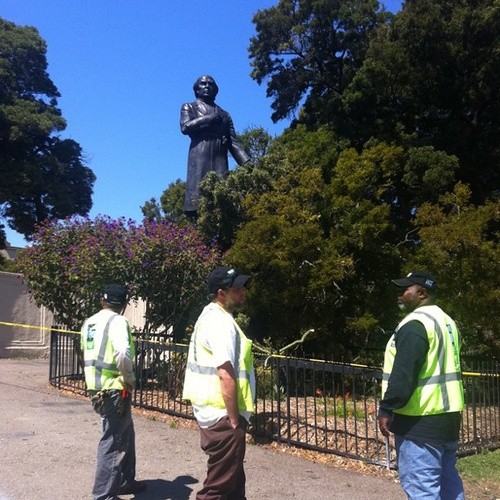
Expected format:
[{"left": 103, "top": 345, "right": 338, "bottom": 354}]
[
  {"left": 99, "top": 494, "right": 125, "bottom": 500},
  {"left": 116, "top": 481, "right": 149, "bottom": 495}
]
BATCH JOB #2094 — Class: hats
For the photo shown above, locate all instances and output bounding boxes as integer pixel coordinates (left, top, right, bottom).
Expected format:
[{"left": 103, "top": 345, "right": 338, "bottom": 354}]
[
  {"left": 390, "top": 271, "right": 438, "bottom": 290},
  {"left": 103, "top": 283, "right": 127, "bottom": 305},
  {"left": 208, "top": 266, "right": 252, "bottom": 294}
]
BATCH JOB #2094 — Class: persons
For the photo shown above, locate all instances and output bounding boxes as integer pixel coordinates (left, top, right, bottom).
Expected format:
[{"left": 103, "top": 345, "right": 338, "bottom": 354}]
[
  {"left": 178, "top": 74, "right": 259, "bottom": 230},
  {"left": 179, "top": 265, "right": 259, "bottom": 500},
  {"left": 373, "top": 267, "right": 469, "bottom": 500},
  {"left": 78, "top": 281, "right": 142, "bottom": 500}
]
